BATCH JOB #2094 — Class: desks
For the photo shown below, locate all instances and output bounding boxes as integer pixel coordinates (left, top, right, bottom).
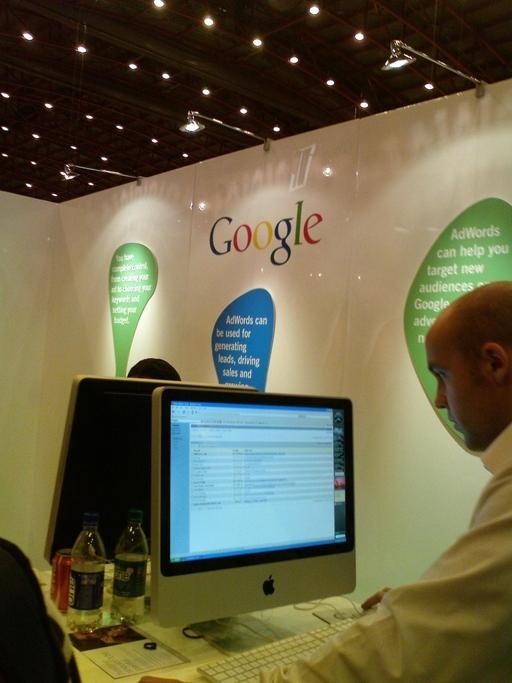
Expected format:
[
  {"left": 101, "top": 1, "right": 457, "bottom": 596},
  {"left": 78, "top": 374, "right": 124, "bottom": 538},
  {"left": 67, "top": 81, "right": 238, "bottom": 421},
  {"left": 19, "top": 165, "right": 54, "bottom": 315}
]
[{"left": 32, "top": 563, "right": 363, "bottom": 682}]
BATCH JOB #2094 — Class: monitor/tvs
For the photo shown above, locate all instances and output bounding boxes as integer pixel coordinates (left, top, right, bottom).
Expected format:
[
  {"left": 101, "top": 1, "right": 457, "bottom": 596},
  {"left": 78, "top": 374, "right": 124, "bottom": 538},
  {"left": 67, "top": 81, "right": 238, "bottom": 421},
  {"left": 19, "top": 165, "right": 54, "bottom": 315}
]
[
  {"left": 43, "top": 373, "right": 259, "bottom": 599},
  {"left": 151, "top": 386, "right": 356, "bottom": 658}
]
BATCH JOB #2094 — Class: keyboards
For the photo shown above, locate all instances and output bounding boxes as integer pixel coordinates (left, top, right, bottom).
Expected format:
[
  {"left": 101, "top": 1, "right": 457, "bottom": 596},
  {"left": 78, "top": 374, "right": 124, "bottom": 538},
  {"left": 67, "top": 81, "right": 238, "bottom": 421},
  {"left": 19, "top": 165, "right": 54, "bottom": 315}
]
[{"left": 196, "top": 618, "right": 372, "bottom": 683}]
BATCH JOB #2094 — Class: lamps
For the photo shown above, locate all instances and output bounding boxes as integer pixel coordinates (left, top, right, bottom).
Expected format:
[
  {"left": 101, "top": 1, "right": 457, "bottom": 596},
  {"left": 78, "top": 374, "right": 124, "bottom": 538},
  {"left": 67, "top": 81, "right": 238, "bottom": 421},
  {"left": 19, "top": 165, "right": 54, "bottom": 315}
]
[
  {"left": 59, "top": 164, "right": 143, "bottom": 186},
  {"left": 380, "top": 39, "right": 489, "bottom": 98},
  {"left": 177, "top": 109, "right": 270, "bottom": 151}
]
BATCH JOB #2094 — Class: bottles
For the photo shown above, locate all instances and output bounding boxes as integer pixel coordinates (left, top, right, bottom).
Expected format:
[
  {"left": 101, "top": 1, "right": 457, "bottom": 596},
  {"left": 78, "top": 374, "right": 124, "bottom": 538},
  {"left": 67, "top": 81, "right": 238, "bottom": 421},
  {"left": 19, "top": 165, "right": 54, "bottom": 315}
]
[
  {"left": 69, "top": 513, "right": 104, "bottom": 635},
  {"left": 110, "top": 507, "right": 149, "bottom": 628}
]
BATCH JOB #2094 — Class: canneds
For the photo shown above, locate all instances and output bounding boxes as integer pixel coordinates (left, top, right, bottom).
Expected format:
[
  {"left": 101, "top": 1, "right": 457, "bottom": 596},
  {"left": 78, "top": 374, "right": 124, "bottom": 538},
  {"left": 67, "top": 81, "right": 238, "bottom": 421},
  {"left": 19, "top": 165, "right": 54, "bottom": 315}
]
[{"left": 50, "top": 548, "right": 73, "bottom": 614}]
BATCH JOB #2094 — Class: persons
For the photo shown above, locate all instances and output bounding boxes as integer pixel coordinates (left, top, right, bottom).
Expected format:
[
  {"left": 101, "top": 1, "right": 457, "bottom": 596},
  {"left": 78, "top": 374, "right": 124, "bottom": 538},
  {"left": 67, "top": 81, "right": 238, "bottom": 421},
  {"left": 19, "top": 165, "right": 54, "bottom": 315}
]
[
  {"left": 135, "top": 279, "right": 511, "bottom": 683},
  {"left": 0, "top": 537, "right": 70, "bottom": 682},
  {"left": 128, "top": 358, "right": 181, "bottom": 381}
]
